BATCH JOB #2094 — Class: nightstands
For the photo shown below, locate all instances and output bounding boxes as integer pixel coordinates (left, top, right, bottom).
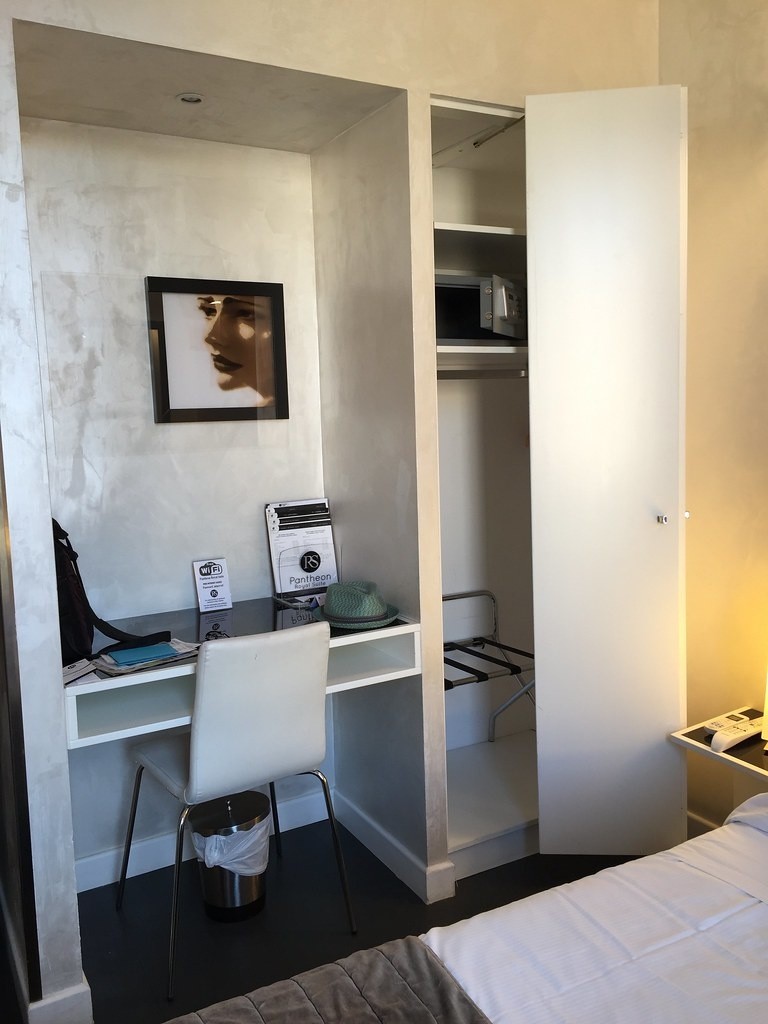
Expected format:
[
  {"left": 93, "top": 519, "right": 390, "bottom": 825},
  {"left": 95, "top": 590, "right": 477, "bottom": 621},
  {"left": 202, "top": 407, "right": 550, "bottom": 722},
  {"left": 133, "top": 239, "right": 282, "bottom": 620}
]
[{"left": 668, "top": 706, "right": 768, "bottom": 810}]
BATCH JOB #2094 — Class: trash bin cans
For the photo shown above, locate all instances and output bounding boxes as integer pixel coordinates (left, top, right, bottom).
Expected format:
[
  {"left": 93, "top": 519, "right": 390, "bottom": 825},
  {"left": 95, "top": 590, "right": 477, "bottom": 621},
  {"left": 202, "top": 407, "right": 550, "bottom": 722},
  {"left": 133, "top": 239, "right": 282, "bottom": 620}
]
[{"left": 188, "top": 789, "right": 274, "bottom": 922}]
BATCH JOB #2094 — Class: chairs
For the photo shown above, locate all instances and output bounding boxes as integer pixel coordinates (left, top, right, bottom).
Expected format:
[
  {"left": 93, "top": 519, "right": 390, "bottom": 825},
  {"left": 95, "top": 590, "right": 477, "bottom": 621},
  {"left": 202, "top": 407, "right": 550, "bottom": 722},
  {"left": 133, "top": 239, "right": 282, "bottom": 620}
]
[{"left": 115, "top": 620, "right": 358, "bottom": 1001}]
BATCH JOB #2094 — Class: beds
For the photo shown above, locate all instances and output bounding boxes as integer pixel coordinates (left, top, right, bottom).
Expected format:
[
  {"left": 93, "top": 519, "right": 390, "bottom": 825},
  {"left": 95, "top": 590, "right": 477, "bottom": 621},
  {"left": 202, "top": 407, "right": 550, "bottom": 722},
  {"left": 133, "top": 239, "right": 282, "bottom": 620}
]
[{"left": 158, "top": 790, "right": 767, "bottom": 1022}]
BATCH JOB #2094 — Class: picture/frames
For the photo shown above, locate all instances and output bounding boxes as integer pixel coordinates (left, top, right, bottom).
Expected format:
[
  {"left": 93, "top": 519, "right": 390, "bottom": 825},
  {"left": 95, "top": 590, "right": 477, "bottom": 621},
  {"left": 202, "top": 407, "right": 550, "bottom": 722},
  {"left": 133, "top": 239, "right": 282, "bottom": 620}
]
[{"left": 144, "top": 276, "right": 289, "bottom": 423}]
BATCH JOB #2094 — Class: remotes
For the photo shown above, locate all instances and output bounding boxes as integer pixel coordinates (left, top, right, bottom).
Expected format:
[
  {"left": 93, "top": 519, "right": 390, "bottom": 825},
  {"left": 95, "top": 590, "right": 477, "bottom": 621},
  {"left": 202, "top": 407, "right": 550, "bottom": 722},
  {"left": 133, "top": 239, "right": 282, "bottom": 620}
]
[
  {"left": 704, "top": 712, "right": 749, "bottom": 734},
  {"left": 711, "top": 716, "right": 763, "bottom": 752}
]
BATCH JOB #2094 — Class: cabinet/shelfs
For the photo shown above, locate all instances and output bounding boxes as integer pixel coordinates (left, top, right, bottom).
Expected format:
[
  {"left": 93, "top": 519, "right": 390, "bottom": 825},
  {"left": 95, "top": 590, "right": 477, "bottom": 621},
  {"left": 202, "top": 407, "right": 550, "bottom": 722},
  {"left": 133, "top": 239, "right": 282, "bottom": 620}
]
[{"left": 425, "top": 82, "right": 689, "bottom": 884}]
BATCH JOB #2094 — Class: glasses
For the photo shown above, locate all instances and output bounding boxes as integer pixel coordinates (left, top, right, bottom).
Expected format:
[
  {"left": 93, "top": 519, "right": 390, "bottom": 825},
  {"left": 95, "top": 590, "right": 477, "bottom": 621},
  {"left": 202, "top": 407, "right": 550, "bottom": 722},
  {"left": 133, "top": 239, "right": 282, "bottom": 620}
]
[{"left": 205, "top": 630, "right": 229, "bottom": 640}]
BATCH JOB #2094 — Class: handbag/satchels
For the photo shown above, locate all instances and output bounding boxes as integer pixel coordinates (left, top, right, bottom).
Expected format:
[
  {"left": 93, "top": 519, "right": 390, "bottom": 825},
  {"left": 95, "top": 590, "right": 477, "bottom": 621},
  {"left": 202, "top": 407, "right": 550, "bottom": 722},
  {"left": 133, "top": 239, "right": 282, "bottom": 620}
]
[{"left": 52, "top": 517, "right": 172, "bottom": 665}]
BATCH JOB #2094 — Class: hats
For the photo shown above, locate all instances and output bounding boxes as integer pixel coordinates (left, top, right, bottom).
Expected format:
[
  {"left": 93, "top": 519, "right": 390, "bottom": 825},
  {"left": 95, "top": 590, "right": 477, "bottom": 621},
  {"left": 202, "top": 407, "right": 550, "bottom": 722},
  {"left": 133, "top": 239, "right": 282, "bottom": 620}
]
[{"left": 312, "top": 578, "right": 399, "bottom": 629}]
